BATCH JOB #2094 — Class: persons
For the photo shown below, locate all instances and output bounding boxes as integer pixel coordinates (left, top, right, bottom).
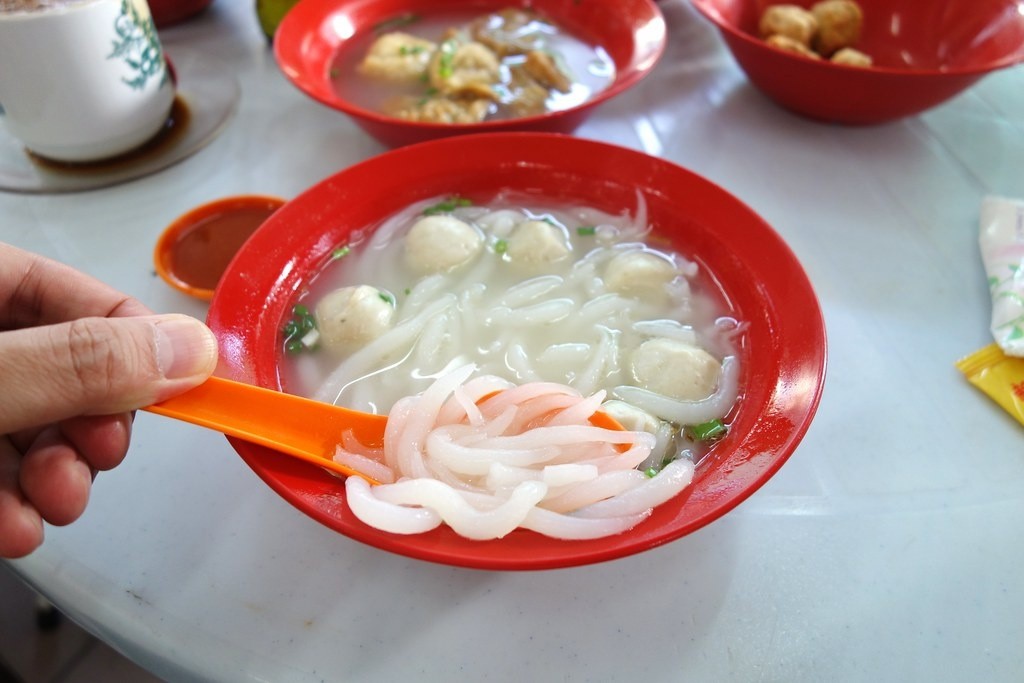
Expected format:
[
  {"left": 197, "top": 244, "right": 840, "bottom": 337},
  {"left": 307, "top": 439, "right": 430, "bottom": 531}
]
[{"left": 1, "top": 241, "right": 217, "bottom": 559}]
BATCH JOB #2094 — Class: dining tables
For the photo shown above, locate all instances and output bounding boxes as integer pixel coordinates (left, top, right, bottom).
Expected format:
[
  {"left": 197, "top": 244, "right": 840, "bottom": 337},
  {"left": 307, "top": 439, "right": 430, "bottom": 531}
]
[{"left": 0, "top": 0, "right": 1024, "bottom": 681}]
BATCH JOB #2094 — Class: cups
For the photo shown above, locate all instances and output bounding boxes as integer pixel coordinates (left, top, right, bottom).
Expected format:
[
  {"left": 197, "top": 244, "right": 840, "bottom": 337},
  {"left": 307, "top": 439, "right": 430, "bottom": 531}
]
[{"left": 0, "top": 1, "right": 174, "bottom": 167}]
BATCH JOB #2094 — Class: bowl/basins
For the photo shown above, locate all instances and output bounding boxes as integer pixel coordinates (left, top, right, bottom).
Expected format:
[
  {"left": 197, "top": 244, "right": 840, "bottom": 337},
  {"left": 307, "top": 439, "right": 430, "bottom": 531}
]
[
  {"left": 272, "top": 1, "right": 668, "bottom": 151},
  {"left": 206, "top": 132, "right": 827, "bottom": 570},
  {"left": 688, "top": 0, "right": 1023, "bottom": 128}
]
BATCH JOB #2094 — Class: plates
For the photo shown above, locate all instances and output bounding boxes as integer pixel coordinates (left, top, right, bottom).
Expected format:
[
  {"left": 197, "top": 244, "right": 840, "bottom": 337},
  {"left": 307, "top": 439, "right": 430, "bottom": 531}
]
[
  {"left": 150, "top": 197, "right": 293, "bottom": 300},
  {"left": 1, "top": 41, "right": 241, "bottom": 193}
]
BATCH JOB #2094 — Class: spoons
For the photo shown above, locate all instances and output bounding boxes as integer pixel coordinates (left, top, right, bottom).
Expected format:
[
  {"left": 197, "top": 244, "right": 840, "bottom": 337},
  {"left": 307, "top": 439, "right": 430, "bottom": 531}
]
[{"left": 135, "top": 375, "right": 638, "bottom": 494}]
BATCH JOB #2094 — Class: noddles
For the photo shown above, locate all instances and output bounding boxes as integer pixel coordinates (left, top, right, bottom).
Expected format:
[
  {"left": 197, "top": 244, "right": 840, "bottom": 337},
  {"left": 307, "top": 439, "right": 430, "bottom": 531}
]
[{"left": 276, "top": 193, "right": 749, "bottom": 543}]
